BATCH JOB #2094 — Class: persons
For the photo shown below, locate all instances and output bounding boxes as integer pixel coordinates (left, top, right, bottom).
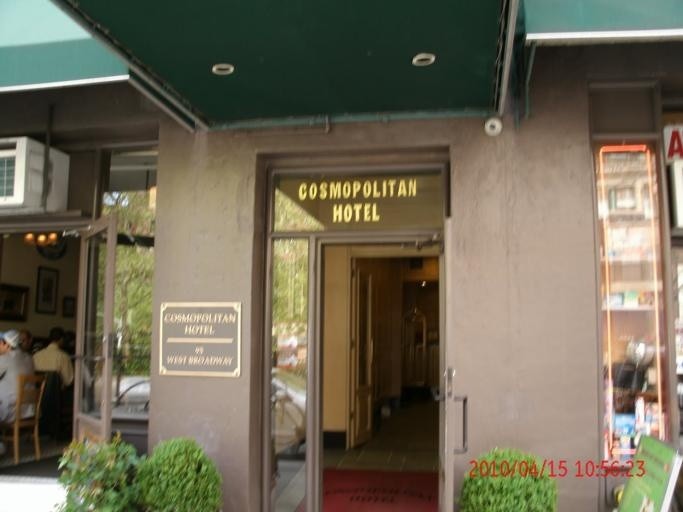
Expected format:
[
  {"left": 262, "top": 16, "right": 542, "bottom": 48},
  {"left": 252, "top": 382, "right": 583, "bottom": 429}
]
[
  {"left": 33, "top": 326, "right": 74, "bottom": 390},
  {"left": 0, "top": 330, "right": 36, "bottom": 456},
  {"left": 18, "top": 330, "right": 44, "bottom": 356}
]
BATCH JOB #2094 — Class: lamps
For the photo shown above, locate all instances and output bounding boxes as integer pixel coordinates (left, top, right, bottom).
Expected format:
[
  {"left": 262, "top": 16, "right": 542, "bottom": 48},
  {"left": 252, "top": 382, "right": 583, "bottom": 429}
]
[
  {"left": 483, "top": 112, "right": 503, "bottom": 138},
  {"left": 23, "top": 233, "right": 59, "bottom": 249}
]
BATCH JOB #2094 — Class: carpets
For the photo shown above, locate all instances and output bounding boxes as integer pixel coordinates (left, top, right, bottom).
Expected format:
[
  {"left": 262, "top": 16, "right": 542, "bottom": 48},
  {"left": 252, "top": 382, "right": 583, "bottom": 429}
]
[{"left": 292, "top": 466, "right": 439, "bottom": 512}]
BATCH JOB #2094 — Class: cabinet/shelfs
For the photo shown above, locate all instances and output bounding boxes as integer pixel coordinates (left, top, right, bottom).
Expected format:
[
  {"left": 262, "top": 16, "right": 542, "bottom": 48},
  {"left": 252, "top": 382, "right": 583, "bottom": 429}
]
[{"left": 597, "top": 139, "right": 669, "bottom": 464}]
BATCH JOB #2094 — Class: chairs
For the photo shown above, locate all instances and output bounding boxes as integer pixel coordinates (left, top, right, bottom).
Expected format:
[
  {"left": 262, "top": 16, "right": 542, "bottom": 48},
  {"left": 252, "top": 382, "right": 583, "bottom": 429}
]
[
  {"left": 0, "top": 374, "right": 48, "bottom": 467},
  {"left": 34, "top": 371, "right": 74, "bottom": 441}
]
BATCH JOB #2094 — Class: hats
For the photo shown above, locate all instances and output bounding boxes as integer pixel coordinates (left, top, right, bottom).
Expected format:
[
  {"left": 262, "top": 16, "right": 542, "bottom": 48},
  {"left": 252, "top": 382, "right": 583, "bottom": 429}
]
[{"left": 1, "top": 329, "right": 21, "bottom": 348}]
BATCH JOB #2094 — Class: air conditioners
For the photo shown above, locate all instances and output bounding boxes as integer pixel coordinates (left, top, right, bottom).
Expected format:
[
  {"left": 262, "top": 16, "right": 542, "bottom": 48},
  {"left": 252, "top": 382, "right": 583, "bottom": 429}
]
[{"left": 0, "top": 136, "right": 71, "bottom": 218}]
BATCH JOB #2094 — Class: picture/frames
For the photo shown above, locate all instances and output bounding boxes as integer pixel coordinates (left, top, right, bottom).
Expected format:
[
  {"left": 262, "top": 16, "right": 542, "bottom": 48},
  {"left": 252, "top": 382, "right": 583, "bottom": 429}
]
[
  {"left": 63, "top": 296, "right": 76, "bottom": 318},
  {"left": 0, "top": 283, "right": 30, "bottom": 322},
  {"left": 35, "top": 266, "right": 60, "bottom": 315}
]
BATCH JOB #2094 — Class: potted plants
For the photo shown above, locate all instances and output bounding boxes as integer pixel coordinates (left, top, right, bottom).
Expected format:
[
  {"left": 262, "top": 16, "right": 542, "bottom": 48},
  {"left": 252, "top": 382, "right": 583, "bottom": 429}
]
[
  {"left": 56, "top": 431, "right": 145, "bottom": 512},
  {"left": 132, "top": 437, "right": 224, "bottom": 512}
]
